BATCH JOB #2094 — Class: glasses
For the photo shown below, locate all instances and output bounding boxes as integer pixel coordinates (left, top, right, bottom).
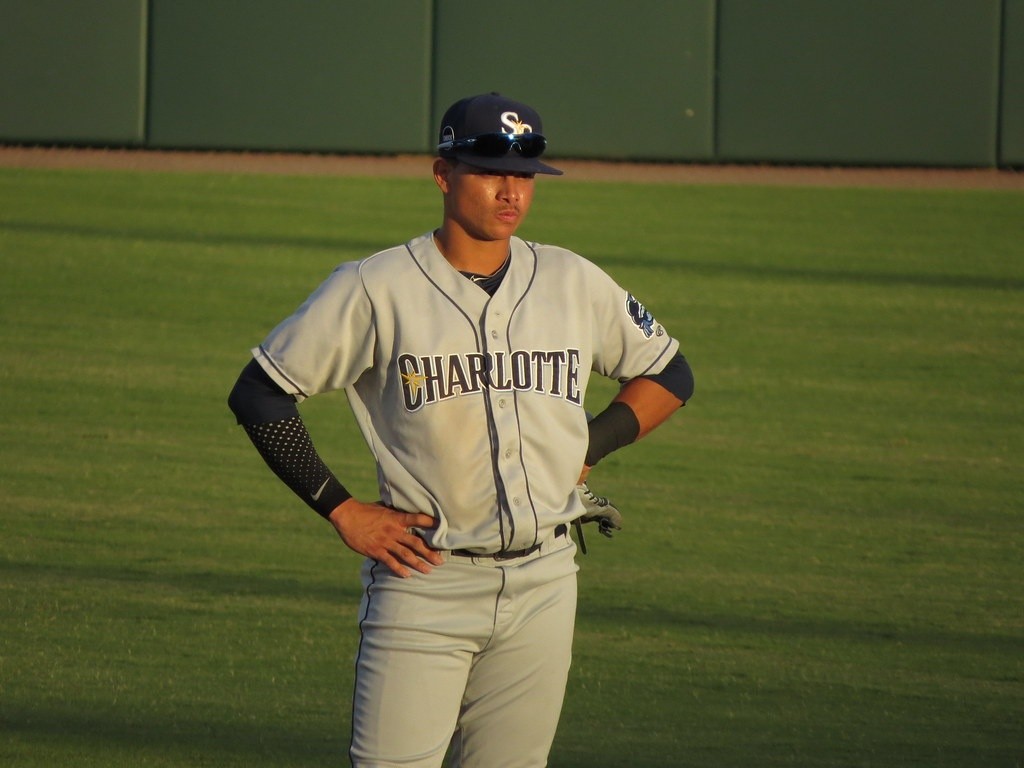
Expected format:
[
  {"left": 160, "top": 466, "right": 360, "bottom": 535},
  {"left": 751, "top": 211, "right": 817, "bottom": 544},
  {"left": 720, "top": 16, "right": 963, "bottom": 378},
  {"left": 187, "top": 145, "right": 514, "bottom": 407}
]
[{"left": 437, "top": 133, "right": 547, "bottom": 160}]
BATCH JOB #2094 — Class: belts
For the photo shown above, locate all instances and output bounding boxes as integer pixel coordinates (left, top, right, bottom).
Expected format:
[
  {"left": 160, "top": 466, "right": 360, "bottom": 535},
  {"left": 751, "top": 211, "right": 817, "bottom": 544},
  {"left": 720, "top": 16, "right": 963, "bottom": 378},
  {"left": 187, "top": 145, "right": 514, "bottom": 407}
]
[{"left": 451, "top": 523, "right": 567, "bottom": 562}]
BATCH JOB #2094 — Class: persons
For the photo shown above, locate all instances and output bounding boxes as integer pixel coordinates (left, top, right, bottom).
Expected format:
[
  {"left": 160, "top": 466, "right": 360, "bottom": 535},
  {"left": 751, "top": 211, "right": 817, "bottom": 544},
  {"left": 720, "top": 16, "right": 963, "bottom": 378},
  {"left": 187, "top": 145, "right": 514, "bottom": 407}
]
[{"left": 228, "top": 91, "right": 694, "bottom": 768}]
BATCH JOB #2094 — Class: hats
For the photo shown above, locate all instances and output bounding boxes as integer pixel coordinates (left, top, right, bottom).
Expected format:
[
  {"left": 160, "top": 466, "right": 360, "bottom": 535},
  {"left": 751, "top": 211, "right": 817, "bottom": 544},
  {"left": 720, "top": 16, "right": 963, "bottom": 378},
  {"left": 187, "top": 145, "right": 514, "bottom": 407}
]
[{"left": 436, "top": 92, "right": 566, "bottom": 175}]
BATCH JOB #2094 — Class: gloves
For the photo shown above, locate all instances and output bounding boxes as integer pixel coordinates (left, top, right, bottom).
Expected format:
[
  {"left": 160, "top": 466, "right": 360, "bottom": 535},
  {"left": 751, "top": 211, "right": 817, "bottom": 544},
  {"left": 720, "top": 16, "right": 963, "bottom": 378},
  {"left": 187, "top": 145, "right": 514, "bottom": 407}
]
[{"left": 577, "top": 479, "right": 622, "bottom": 538}]
